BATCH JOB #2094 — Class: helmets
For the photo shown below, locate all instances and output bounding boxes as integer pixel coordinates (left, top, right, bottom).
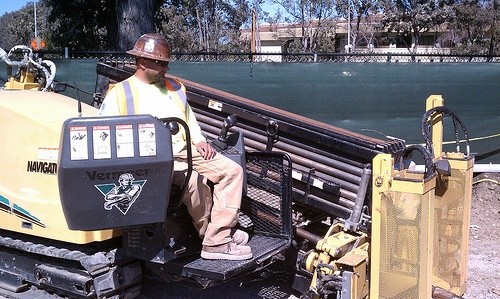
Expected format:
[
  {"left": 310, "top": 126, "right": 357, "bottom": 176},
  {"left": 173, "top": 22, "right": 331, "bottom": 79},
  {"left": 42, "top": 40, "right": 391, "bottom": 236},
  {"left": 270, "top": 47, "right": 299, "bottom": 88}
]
[{"left": 126, "top": 33, "right": 174, "bottom": 62}]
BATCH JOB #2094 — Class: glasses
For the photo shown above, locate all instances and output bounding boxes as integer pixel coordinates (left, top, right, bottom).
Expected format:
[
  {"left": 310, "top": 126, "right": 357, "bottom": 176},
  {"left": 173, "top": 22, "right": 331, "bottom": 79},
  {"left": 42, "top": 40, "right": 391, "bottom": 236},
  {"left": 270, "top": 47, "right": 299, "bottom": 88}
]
[{"left": 144, "top": 58, "right": 169, "bottom": 67}]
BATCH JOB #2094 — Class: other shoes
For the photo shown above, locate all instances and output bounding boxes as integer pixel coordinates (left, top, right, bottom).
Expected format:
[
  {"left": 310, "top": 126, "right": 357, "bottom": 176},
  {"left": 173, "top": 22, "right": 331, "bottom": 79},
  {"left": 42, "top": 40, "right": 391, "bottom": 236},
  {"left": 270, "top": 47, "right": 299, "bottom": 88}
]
[
  {"left": 201, "top": 242, "right": 253, "bottom": 260},
  {"left": 230, "top": 229, "right": 249, "bottom": 246}
]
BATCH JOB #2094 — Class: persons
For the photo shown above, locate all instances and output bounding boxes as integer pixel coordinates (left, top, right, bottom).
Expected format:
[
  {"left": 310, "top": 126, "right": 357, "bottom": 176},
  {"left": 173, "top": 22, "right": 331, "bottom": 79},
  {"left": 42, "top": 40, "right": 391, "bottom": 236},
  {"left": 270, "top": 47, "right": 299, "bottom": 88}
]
[{"left": 101, "top": 34, "right": 252, "bottom": 261}]
